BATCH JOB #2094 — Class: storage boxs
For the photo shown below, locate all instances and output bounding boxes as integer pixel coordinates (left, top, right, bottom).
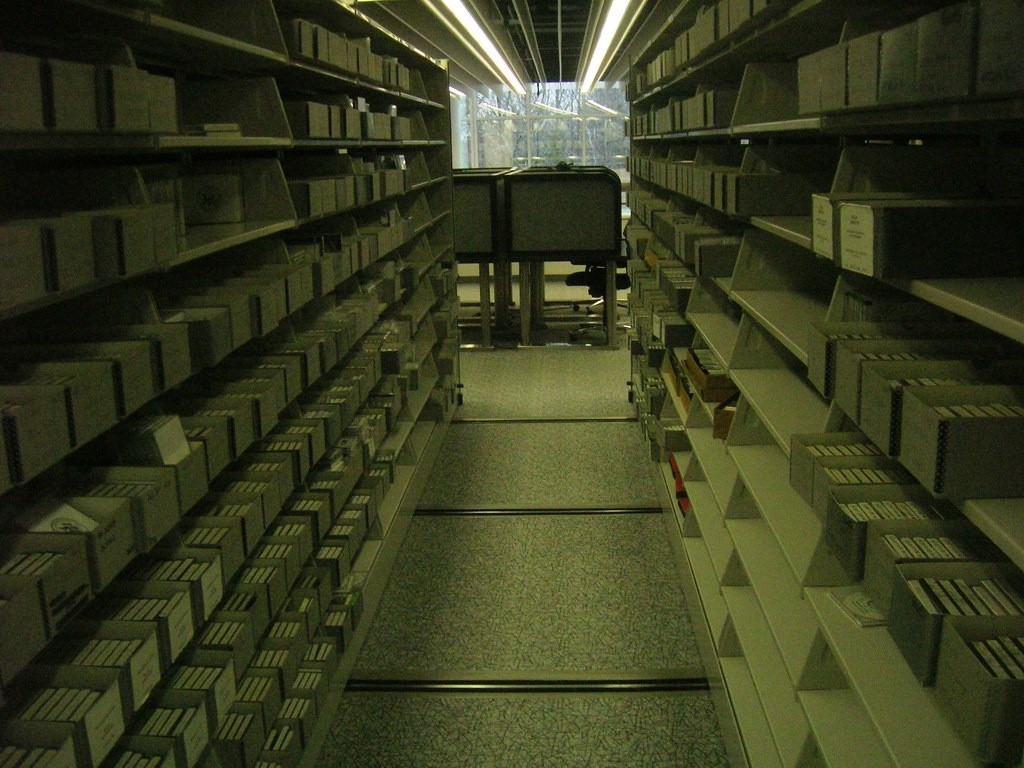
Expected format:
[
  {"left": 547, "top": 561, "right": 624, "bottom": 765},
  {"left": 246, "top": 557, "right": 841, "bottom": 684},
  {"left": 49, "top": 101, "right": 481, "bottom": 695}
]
[{"left": 0, "top": 0, "right": 1024, "bottom": 768}]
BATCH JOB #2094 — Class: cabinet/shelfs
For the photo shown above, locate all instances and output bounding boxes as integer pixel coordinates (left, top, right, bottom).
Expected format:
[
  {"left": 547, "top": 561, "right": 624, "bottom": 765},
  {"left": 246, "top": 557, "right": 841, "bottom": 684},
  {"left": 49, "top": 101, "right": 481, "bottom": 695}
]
[
  {"left": 629, "top": 0, "right": 1024, "bottom": 768},
  {"left": 0, "top": 0, "right": 465, "bottom": 768}
]
